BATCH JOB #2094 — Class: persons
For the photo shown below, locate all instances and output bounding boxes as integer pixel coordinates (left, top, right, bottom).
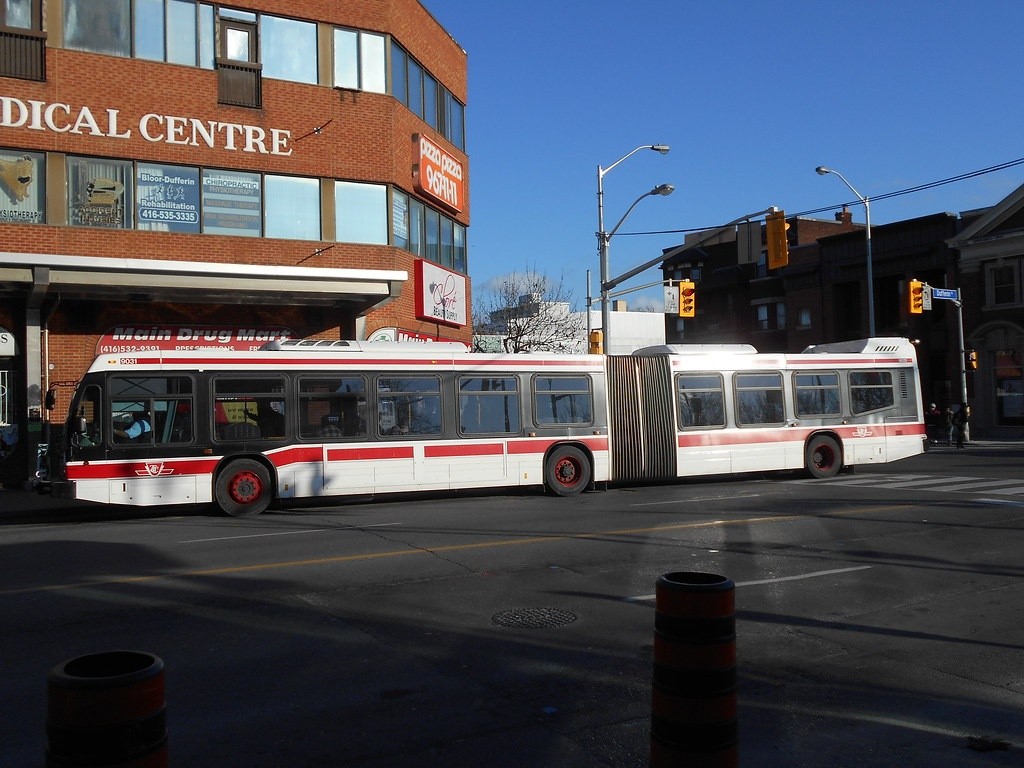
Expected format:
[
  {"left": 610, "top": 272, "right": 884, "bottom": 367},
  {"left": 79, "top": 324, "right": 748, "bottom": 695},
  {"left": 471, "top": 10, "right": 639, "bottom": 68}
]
[
  {"left": 941, "top": 404, "right": 956, "bottom": 447},
  {"left": 244, "top": 400, "right": 286, "bottom": 439},
  {"left": 926, "top": 402, "right": 941, "bottom": 444},
  {"left": 113, "top": 411, "right": 152, "bottom": 444},
  {"left": 952, "top": 402, "right": 970, "bottom": 450}
]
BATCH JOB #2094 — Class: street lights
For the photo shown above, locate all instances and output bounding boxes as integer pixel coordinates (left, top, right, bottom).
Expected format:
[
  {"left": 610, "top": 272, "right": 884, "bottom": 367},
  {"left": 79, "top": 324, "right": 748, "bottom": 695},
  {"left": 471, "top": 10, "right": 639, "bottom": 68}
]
[
  {"left": 595, "top": 143, "right": 676, "bottom": 356},
  {"left": 815, "top": 166, "right": 875, "bottom": 338}
]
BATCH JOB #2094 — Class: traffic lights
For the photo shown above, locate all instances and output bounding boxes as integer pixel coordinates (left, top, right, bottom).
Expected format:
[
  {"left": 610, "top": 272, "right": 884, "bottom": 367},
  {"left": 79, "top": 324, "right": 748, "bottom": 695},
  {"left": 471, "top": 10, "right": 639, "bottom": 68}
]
[
  {"left": 908, "top": 280, "right": 924, "bottom": 315},
  {"left": 679, "top": 282, "right": 695, "bottom": 318},
  {"left": 766, "top": 209, "right": 790, "bottom": 269}
]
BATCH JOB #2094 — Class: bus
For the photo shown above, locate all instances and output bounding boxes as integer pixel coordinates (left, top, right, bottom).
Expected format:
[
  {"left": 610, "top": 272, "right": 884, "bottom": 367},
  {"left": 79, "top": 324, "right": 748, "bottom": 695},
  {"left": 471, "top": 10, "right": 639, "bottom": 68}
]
[{"left": 46, "top": 337, "right": 929, "bottom": 519}]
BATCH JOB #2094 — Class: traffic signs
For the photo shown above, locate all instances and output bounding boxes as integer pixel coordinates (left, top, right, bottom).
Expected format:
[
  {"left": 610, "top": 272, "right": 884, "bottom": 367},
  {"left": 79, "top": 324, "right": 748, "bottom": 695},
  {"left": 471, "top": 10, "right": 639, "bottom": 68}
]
[
  {"left": 922, "top": 286, "right": 932, "bottom": 311},
  {"left": 663, "top": 286, "right": 679, "bottom": 314}
]
